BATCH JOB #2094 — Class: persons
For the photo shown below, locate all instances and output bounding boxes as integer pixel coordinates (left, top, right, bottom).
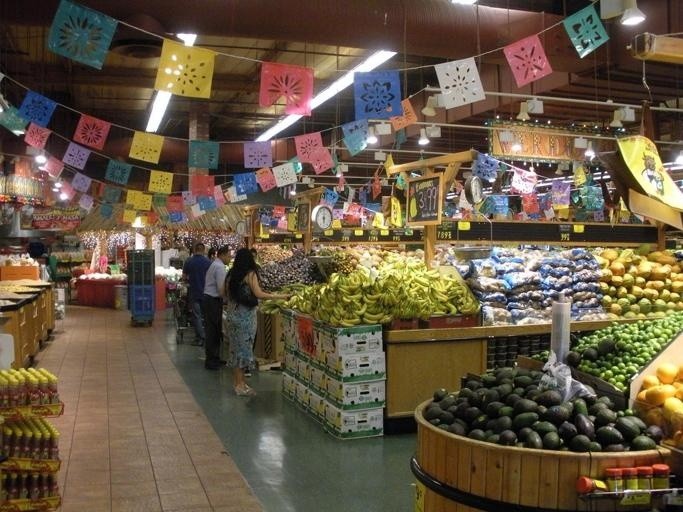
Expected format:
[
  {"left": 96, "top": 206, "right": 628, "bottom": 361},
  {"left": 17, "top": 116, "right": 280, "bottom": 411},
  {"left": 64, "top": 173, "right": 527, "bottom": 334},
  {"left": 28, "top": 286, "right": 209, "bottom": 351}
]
[
  {"left": 207, "top": 247, "right": 217, "bottom": 260},
  {"left": 98, "top": 251, "right": 107, "bottom": 273},
  {"left": 28, "top": 237, "right": 48, "bottom": 257},
  {"left": 180, "top": 242, "right": 212, "bottom": 346},
  {"left": 223, "top": 249, "right": 292, "bottom": 397},
  {"left": 201, "top": 245, "right": 232, "bottom": 371},
  {"left": 249, "top": 248, "right": 261, "bottom": 270}
]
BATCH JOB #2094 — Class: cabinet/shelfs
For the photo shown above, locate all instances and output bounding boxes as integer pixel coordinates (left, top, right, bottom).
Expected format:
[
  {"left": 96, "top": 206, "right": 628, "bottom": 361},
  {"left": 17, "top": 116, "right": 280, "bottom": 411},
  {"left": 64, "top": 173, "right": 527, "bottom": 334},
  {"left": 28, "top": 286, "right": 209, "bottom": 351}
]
[{"left": 48, "top": 254, "right": 92, "bottom": 304}]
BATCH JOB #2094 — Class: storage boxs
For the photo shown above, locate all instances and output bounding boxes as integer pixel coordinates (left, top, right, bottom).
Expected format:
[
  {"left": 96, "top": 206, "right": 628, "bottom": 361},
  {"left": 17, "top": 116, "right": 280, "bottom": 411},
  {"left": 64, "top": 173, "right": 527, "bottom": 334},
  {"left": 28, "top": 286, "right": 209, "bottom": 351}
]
[{"left": 281, "top": 308, "right": 386, "bottom": 441}]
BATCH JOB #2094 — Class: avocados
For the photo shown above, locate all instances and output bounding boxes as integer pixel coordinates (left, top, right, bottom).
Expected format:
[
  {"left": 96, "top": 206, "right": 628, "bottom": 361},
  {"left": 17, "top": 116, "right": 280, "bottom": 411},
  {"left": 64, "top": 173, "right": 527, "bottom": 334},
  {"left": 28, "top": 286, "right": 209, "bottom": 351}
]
[{"left": 425, "top": 365, "right": 662, "bottom": 452}]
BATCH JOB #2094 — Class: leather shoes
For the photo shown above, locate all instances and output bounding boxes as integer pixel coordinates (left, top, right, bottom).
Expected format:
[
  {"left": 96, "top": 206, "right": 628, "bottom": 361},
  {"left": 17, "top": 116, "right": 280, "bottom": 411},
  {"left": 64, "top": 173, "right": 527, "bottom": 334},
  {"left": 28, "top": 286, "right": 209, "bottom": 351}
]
[{"left": 205, "top": 360, "right": 226, "bottom": 370}]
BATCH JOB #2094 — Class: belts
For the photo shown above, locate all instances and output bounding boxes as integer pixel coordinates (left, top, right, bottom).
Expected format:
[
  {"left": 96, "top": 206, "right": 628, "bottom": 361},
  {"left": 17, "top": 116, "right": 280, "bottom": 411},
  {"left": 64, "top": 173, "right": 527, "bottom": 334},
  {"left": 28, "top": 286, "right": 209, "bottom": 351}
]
[{"left": 215, "top": 298, "right": 223, "bottom": 302}]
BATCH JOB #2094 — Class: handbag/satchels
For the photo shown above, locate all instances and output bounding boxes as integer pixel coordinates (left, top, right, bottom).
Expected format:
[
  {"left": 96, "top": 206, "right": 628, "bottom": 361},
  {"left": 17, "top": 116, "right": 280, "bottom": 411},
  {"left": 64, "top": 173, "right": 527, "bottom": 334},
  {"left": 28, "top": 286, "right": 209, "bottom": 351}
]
[{"left": 246, "top": 295, "right": 258, "bottom": 307}]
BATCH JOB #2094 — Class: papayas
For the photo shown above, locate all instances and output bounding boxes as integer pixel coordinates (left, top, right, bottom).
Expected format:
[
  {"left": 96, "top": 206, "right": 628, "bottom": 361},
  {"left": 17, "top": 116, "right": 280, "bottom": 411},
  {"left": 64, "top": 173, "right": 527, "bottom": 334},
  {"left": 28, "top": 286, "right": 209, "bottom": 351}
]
[{"left": 593, "top": 249, "right": 683, "bottom": 319}]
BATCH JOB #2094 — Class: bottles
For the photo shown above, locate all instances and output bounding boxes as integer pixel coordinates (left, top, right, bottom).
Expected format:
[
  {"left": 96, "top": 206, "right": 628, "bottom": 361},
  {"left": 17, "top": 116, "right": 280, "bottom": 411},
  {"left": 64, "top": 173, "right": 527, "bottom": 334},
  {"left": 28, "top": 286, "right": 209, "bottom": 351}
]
[{"left": 0, "top": 366, "right": 61, "bottom": 503}]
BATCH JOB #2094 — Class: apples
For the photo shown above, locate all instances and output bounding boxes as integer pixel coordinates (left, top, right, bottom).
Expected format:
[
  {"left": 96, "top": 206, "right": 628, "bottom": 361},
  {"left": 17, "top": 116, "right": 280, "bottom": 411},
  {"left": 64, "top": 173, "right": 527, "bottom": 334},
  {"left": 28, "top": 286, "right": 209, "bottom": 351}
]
[{"left": 370, "top": 247, "right": 425, "bottom": 264}]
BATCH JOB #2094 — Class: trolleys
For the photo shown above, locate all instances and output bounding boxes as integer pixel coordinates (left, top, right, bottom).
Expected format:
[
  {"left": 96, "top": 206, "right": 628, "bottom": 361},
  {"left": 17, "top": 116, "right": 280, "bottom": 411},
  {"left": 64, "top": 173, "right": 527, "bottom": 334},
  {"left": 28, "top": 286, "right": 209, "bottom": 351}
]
[{"left": 172, "top": 277, "right": 205, "bottom": 345}]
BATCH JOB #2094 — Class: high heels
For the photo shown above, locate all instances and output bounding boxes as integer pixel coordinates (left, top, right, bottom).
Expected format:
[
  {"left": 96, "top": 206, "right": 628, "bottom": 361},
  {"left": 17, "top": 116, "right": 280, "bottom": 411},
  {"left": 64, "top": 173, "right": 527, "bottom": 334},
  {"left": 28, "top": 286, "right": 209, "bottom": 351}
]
[{"left": 231, "top": 383, "right": 257, "bottom": 396}]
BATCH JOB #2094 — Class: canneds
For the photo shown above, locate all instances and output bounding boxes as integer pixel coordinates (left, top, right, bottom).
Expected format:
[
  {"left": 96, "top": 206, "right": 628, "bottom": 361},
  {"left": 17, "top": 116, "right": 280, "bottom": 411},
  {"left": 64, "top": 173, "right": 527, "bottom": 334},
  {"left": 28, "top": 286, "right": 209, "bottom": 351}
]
[{"left": 576, "top": 464, "right": 670, "bottom": 496}]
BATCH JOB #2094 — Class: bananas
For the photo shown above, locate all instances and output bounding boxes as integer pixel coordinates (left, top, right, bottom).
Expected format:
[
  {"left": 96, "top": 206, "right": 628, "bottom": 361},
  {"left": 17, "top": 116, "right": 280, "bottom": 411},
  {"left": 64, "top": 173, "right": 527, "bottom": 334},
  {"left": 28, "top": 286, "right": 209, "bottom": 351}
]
[
  {"left": 260, "top": 264, "right": 482, "bottom": 327},
  {"left": 283, "top": 335, "right": 343, "bottom": 429}
]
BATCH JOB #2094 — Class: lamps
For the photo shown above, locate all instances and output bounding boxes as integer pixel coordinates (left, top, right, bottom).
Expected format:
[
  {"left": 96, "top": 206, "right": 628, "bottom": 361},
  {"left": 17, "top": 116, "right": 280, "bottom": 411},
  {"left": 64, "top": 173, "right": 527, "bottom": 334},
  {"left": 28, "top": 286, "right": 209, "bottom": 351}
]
[
  {"left": 619, "top": 0, "right": 681, "bottom": 65},
  {"left": 145, "top": 31, "right": 397, "bottom": 143}
]
